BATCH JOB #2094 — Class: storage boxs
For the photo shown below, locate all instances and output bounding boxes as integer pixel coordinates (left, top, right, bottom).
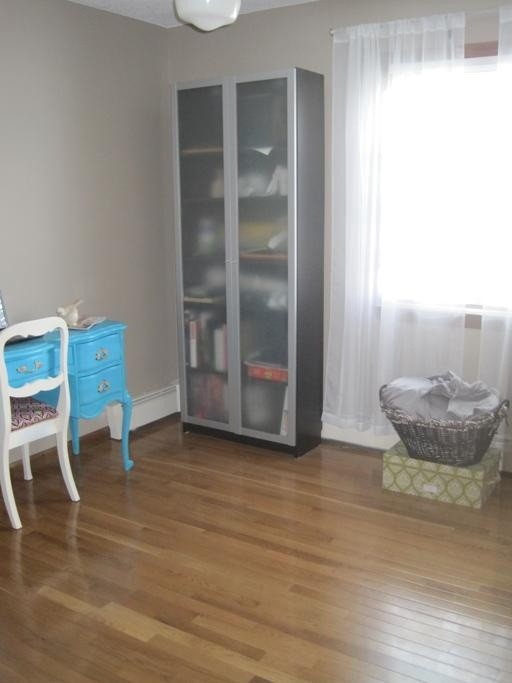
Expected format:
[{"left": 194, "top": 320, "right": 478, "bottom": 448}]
[{"left": 385, "top": 436, "right": 503, "bottom": 510}]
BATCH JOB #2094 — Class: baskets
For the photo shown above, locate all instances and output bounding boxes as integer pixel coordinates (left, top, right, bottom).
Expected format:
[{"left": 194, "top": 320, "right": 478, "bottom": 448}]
[{"left": 377, "top": 382, "right": 510, "bottom": 468}]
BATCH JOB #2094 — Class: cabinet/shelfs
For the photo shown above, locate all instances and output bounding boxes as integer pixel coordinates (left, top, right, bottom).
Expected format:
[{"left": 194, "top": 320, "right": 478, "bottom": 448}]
[
  {"left": 167, "top": 69, "right": 297, "bottom": 459},
  {"left": 5, "top": 334, "right": 72, "bottom": 388},
  {"left": 68, "top": 325, "right": 136, "bottom": 475}
]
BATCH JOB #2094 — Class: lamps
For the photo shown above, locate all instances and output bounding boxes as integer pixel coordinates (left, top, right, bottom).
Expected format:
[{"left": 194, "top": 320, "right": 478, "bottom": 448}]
[{"left": 173, "top": 0, "right": 242, "bottom": 32}]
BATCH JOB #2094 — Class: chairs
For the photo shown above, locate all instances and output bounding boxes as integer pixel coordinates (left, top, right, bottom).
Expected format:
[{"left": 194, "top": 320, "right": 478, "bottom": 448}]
[{"left": 2, "top": 315, "right": 84, "bottom": 531}]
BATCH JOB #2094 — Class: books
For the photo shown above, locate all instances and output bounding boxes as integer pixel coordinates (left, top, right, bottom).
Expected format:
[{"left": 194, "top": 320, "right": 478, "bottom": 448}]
[{"left": 183, "top": 305, "right": 255, "bottom": 374}]
